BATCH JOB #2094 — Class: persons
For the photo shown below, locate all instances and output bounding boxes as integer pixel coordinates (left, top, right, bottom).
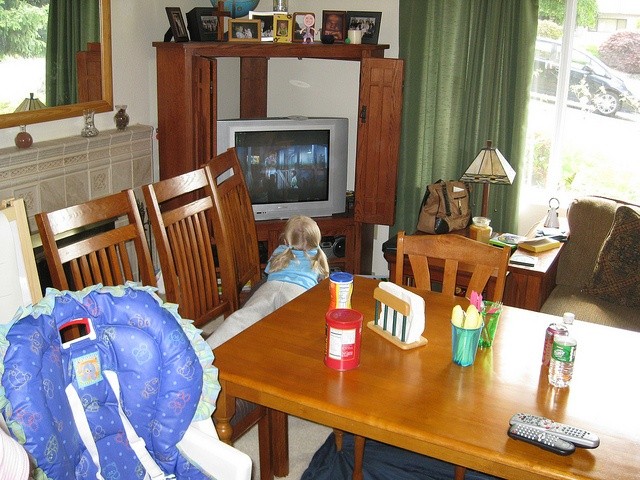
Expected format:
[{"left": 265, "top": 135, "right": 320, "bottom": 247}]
[
  {"left": 299, "top": 14, "right": 318, "bottom": 44},
  {"left": 260, "top": 21, "right": 272, "bottom": 37},
  {"left": 350, "top": 18, "right": 375, "bottom": 37},
  {"left": 278, "top": 23, "right": 288, "bottom": 36},
  {"left": 174, "top": 14, "right": 186, "bottom": 35},
  {"left": 291, "top": 169, "right": 300, "bottom": 199},
  {"left": 205, "top": 215, "right": 329, "bottom": 351},
  {"left": 234, "top": 26, "right": 246, "bottom": 38},
  {"left": 243, "top": 28, "right": 253, "bottom": 38}
]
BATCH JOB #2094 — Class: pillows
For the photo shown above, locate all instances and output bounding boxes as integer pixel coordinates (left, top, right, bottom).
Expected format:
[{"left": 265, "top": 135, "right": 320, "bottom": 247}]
[{"left": 588, "top": 207, "right": 640, "bottom": 307}]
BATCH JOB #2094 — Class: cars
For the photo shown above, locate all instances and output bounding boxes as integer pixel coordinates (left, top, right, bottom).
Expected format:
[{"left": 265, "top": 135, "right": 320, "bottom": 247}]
[{"left": 529, "top": 38, "right": 636, "bottom": 116}]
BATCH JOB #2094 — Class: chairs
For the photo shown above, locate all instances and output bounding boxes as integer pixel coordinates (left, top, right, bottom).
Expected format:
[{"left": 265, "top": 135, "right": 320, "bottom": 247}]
[
  {"left": 0, "top": 282, "right": 253, "bottom": 479},
  {"left": 142, "top": 164, "right": 238, "bottom": 339},
  {"left": 394, "top": 229, "right": 511, "bottom": 302},
  {"left": 35, "top": 187, "right": 161, "bottom": 293},
  {"left": 201, "top": 149, "right": 269, "bottom": 319},
  {"left": 0, "top": 195, "right": 43, "bottom": 323}
]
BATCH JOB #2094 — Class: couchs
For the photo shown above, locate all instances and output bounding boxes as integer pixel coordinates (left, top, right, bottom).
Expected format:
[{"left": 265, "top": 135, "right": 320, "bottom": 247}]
[{"left": 508, "top": 195, "right": 640, "bottom": 331}]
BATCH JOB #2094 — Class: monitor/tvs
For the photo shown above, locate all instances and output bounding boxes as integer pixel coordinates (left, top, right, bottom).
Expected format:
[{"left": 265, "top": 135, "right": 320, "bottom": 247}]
[{"left": 216, "top": 117, "right": 349, "bottom": 222}]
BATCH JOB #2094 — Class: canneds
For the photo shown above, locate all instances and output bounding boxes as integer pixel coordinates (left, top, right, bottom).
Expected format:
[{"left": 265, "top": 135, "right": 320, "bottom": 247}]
[{"left": 541, "top": 322, "right": 570, "bottom": 368}]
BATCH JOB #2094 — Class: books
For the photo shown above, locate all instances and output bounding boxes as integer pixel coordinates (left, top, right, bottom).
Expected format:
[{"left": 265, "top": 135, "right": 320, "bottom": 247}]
[
  {"left": 517, "top": 236, "right": 560, "bottom": 252},
  {"left": 489, "top": 233, "right": 528, "bottom": 249}
]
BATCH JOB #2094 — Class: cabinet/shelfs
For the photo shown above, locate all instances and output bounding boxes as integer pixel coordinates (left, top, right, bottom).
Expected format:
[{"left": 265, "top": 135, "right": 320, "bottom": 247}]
[
  {"left": 151, "top": 39, "right": 403, "bottom": 274},
  {"left": 75, "top": 41, "right": 101, "bottom": 101}
]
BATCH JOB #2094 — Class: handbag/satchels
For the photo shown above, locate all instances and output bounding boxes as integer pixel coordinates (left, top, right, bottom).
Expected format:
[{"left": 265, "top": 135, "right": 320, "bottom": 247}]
[{"left": 416, "top": 179, "right": 473, "bottom": 234}]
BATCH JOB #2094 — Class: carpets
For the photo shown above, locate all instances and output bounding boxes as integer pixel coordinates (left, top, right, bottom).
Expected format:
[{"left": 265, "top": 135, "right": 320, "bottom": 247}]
[{"left": 300, "top": 427, "right": 493, "bottom": 480}]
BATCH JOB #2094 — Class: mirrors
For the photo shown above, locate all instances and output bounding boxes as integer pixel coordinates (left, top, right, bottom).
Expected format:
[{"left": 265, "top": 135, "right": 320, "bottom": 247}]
[{"left": 0, "top": 0, "right": 114, "bottom": 130}]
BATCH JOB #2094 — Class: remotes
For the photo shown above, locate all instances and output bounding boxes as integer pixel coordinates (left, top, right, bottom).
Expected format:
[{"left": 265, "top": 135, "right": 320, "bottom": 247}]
[
  {"left": 507, "top": 423, "right": 576, "bottom": 457},
  {"left": 509, "top": 413, "right": 600, "bottom": 449}
]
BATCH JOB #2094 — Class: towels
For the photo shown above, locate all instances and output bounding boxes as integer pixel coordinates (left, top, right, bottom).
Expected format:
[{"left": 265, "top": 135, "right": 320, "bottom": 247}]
[{"left": 0, "top": 215, "right": 32, "bottom": 323}]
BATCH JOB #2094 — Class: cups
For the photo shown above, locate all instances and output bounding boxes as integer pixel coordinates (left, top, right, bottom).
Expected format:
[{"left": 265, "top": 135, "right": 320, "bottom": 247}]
[
  {"left": 475, "top": 300, "right": 499, "bottom": 346},
  {"left": 450, "top": 312, "right": 483, "bottom": 366}
]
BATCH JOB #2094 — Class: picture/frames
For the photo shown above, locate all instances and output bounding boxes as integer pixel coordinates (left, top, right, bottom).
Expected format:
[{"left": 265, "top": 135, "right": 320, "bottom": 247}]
[
  {"left": 274, "top": 14, "right": 292, "bottom": 42},
  {"left": 248, "top": 10, "right": 287, "bottom": 41},
  {"left": 344, "top": 9, "right": 383, "bottom": 44},
  {"left": 164, "top": 6, "right": 189, "bottom": 41},
  {"left": 321, "top": 9, "right": 346, "bottom": 43},
  {"left": 227, "top": 19, "right": 262, "bottom": 42},
  {"left": 197, "top": 9, "right": 233, "bottom": 42},
  {"left": 292, "top": 11, "right": 315, "bottom": 42}
]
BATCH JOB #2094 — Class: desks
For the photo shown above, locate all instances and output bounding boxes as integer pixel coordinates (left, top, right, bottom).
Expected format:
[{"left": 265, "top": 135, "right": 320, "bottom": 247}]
[
  {"left": 204, "top": 277, "right": 639, "bottom": 480},
  {"left": 383, "top": 222, "right": 518, "bottom": 304}
]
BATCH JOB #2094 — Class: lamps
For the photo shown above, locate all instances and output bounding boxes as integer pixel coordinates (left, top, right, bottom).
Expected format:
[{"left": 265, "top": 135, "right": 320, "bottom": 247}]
[
  {"left": 459, "top": 140, "right": 517, "bottom": 217},
  {"left": 14, "top": 93, "right": 48, "bottom": 114}
]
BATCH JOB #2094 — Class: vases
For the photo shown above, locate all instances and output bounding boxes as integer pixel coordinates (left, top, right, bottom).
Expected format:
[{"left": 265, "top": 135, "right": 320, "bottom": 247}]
[
  {"left": 81, "top": 108, "right": 99, "bottom": 137},
  {"left": 15, "top": 125, "right": 33, "bottom": 148},
  {"left": 115, "top": 105, "right": 130, "bottom": 129}
]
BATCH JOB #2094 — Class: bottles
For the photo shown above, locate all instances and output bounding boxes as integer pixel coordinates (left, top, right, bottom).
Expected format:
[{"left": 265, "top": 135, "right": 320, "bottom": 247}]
[
  {"left": 324, "top": 310, "right": 362, "bottom": 372},
  {"left": 324, "top": 272, "right": 354, "bottom": 325},
  {"left": 348, "top": 29, "right": 361, "bottom": 44},
  {"left": 470, "top": 216, "right": 493, "bottom": 244},
  {"left": 272, "top": 0, "right": 287, "bottom": 11},
  {"left": 548, "top": 311, "right": 575, "bottom": 387}
]
